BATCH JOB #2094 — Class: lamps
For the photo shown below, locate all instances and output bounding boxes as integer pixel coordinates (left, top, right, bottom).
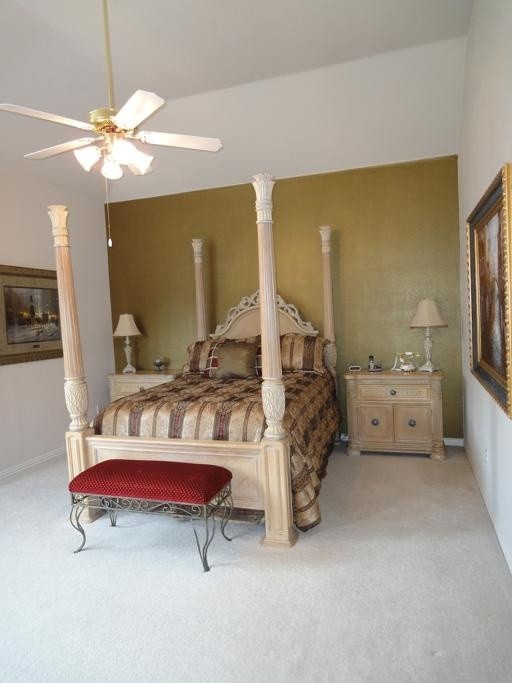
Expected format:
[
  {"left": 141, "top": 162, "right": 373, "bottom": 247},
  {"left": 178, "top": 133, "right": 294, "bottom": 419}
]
[
  {"left": 112, "top": 313, "right": 142, "bottom": 374},
  {"left": 409, "top": 298, "right": 448, "bottom": 372},
  {"left": 73, "top": 134, "right": 154, "bottom": 180}
]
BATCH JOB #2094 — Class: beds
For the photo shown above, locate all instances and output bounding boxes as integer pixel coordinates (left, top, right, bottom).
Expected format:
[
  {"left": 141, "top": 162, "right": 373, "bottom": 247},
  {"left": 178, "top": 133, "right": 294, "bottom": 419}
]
[{"left": 46, "top": 171, "right": 342, "bottom": 550}]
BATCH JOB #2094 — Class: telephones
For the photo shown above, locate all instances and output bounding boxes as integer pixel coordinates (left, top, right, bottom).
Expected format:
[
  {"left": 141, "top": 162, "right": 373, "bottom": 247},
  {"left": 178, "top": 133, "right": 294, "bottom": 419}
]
[
  {"left": 367, "top": 352, "right": 382, "bottom": 371},
  {"left": 395, "top": 351, "right": 422, "bottom": 371}
]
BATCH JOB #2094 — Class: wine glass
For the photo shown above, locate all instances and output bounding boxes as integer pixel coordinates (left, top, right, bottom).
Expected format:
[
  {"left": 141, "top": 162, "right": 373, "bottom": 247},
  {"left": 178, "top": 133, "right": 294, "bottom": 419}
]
[{"left": 154, "top": 361, "right": 163, "bottom": 371}]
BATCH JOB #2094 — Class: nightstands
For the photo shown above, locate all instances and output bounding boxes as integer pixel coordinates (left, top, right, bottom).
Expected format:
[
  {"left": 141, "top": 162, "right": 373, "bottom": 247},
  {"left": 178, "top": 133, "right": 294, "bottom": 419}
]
[
  {"left": 104, "top": 369, "right": 182, "bottom": 403},
  {"left": 343, "top": 368, "right": 447, "bottom": 462}
]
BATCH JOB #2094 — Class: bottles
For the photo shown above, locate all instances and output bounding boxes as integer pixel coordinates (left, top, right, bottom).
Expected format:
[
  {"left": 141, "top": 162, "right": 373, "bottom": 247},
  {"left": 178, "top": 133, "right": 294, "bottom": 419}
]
[{"left": 369, "top": 356, "right": 374, "bottom": 369}]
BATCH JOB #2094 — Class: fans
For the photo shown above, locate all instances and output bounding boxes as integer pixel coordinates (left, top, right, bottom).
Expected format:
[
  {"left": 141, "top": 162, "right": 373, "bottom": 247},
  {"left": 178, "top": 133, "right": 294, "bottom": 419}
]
[{"left": 0, "top": 0, "right": 223, "bottom": 177}]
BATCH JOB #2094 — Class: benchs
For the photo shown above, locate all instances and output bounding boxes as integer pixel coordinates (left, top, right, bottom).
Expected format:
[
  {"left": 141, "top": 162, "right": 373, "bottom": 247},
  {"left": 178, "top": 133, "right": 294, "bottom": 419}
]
[{"left": 67, "top": 459, "right": 234, "bottom": 572}]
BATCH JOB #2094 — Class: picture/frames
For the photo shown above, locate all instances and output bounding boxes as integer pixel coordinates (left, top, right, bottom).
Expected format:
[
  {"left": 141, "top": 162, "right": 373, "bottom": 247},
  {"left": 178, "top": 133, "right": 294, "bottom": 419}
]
[
  {"left": 0, "top": 265, "right": 63, "bottom": 366},
  {"left": 465, "top": 162, "right": 512, "bottom": 420}
]
